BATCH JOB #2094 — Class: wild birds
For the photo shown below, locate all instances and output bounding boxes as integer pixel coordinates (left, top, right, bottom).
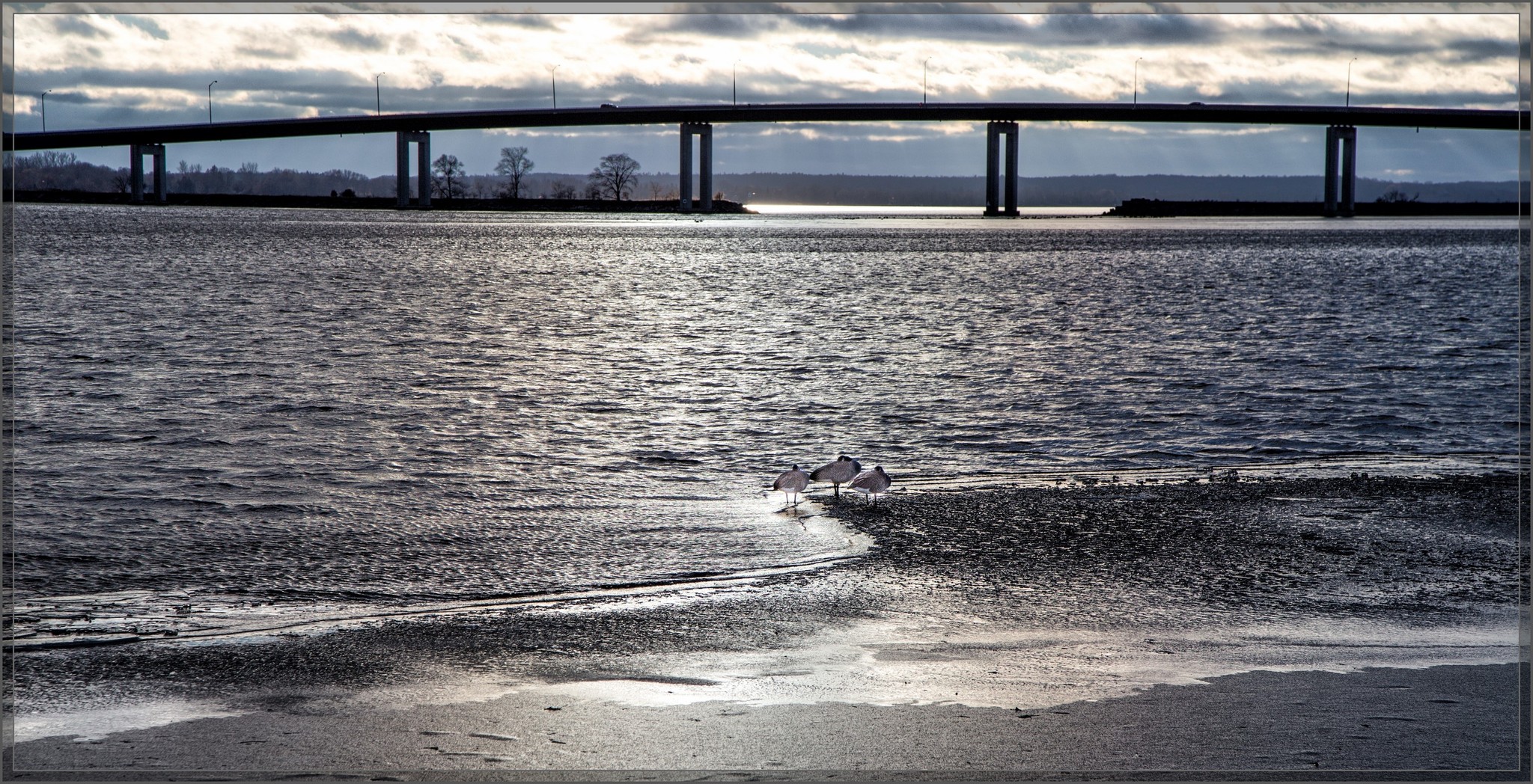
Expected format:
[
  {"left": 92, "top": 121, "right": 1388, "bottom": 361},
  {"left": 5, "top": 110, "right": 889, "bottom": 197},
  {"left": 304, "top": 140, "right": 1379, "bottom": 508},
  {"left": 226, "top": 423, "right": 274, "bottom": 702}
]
[
  {"left": 847, "top": 465, "right": 891, "bottom": 505},
  {"left": 809, "top": 455, "right": 861, "bottom": 498},
  {"left": 773, "top": 465, "right": 810, "bottom": 504}
]
